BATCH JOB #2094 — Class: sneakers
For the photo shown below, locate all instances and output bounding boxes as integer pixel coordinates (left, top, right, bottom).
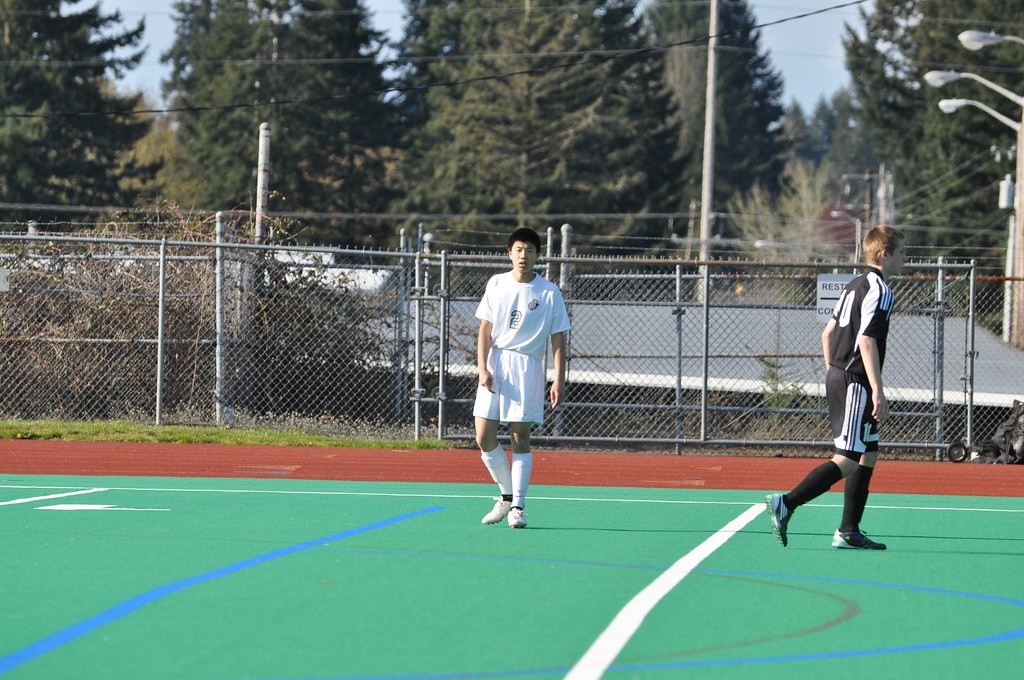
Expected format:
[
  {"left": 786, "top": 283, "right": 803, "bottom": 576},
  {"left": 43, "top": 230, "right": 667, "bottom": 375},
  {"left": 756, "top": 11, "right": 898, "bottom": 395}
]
[
  {"left": 765, "top": 493, "right": 793, "bottom": 547},
  {"left": 832, "top": 529, "right": 887, "bottom": 550},
  {"left": 508, "top": 508, "right": 527, "bottom": 529},
  {"left": 482, "top": 501, "right": 511, "bottom": 525}
]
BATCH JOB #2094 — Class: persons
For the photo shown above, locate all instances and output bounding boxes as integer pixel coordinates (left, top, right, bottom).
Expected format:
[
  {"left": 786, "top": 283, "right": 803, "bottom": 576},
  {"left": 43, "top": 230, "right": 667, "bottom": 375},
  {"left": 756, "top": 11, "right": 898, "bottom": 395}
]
[
  {"left": 472, "top": 228, "right": 573, "bottom": 528},
  {"left": 765, "top": 225, "right": 907, "bottom": 551}
]
